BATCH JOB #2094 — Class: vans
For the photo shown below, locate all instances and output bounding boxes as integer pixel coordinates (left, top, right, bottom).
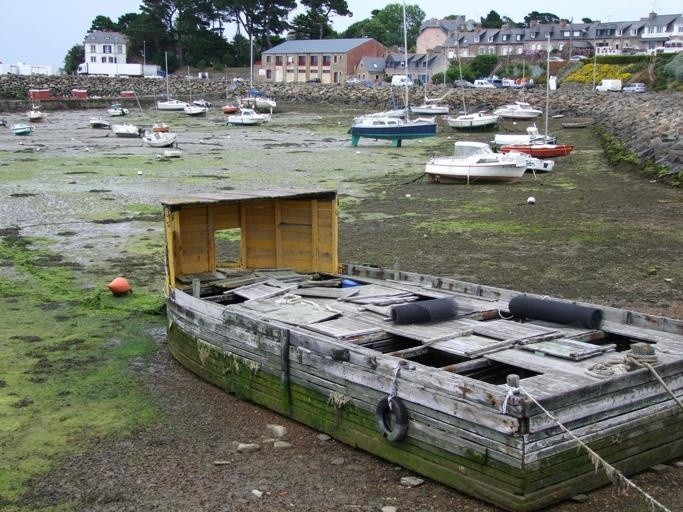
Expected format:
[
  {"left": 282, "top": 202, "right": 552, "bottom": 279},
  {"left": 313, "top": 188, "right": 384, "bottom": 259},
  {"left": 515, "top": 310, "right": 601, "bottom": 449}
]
[{"left": 595, "top": 79, "right": 646, "bottom": 93}]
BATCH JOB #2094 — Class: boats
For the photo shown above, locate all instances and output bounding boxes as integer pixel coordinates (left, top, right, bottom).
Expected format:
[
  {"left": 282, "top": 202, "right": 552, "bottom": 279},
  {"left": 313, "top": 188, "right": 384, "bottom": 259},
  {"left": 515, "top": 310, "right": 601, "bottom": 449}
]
[
  {"left": 29, "top": 106, "right": 45, "bottom": 120},
  {"left": 223, "top": 34, "right": 277, "bottom": 125},
  {"left": 89, "top": 52, "right": 212, "bottom": 147},
  {"left": 163, "top": 263, "right": 683, "bottom": 511},
  {"left": 11, "top": 123, "right": 35, "bottom": 136}
]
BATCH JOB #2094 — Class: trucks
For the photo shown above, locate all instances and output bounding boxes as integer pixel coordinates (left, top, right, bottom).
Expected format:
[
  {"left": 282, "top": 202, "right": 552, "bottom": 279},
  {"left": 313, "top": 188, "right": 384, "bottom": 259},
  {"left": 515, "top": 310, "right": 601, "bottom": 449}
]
[{"left": 77, "top": 62, "right": 141, "bottom": 76}]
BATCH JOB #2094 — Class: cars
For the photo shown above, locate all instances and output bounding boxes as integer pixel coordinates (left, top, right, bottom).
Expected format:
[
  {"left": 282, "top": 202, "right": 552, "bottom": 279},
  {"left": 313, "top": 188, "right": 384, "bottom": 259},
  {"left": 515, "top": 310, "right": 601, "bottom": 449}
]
[{"left": 570, "top": 55, "right": 588, "bottom": 61}]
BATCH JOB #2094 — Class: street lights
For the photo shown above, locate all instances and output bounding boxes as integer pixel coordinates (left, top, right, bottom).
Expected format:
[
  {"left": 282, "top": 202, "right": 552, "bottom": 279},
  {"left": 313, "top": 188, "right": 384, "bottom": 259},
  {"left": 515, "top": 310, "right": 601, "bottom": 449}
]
[{"left": 582, "top": 29, "right": 596, "bottom": 90}]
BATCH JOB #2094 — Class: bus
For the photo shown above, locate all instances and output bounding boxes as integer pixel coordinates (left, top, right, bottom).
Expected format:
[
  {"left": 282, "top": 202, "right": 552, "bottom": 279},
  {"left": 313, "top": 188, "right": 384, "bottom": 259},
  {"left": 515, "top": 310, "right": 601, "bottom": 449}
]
[{"left": 664, "top": 40, "right": 683, "bottom": 54}]
